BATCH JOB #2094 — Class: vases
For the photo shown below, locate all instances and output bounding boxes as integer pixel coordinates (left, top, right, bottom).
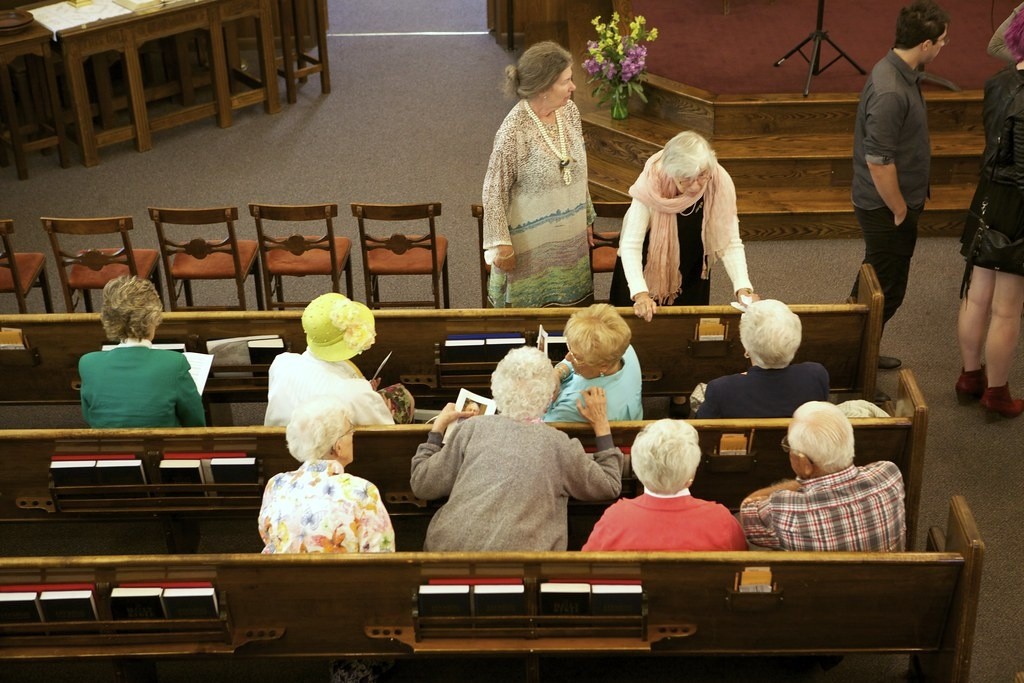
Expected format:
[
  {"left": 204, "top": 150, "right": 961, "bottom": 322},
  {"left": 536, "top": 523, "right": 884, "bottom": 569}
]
[{"left": 609, "top": 80, "right": 630, "bottom": 121}]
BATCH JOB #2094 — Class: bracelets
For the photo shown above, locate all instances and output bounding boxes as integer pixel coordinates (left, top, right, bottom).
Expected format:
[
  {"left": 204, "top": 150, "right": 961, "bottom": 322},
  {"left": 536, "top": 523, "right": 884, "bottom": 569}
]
[
  {"left": 554, "top": 366, "right": 566, "bottom": 378},
  {"left": 497, "top": 250, "right": 514, "bottom": 260}
]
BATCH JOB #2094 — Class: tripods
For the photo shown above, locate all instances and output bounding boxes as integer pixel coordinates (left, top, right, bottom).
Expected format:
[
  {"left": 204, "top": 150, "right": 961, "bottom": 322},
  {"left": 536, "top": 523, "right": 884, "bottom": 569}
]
[{"left": 773, "top": 0, "right": 866, "bottom": 98}]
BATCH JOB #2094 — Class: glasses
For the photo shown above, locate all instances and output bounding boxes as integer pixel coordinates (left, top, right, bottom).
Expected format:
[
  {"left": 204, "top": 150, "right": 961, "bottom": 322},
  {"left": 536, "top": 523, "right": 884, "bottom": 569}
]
[
  {"left": 331, "top": 419, "right": 357, "bottom": 453},
  {"left": 781, "top": 435, "right": 813, "bottom": 464},
  {"left": 932, "top": 38, "right": 949, "bottom": 46},
  {"left": 676, "top": 170, "right": 712, "bottom": 185},
  {"left": 565, "top": 339, "right": 586, "bottom": 368}
]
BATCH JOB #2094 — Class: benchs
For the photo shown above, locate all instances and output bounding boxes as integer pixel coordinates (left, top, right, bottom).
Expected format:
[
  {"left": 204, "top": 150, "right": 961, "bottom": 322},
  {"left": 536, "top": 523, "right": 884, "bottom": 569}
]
[
  {"left": 0, "top": 368, "right": 932, "bottom": 558},
  {"left": 0, "top": 497, "right": 989, "bottom": 683},
  {"left": 0, "top": 263, "right": 887, "bottom": 409}
]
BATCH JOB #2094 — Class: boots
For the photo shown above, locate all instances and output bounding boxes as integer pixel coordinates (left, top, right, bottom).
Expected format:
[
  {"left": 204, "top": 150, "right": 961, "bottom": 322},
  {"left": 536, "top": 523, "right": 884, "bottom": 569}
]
[
  {"left": 980, "top": 382, "right": 1024, "bottom": 423},
  {"left": 957, "top": 364, "right": 986, "bottom": 402}
]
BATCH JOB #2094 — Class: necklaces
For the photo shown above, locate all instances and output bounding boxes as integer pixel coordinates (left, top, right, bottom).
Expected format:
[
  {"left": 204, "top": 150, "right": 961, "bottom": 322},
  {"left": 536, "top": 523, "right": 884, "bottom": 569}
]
[
  {"left": 523, "top": 99, "right": 572, "bottom": 187},
  {"left": 680, "top": 202, "right": 696, "bottom": 216}
]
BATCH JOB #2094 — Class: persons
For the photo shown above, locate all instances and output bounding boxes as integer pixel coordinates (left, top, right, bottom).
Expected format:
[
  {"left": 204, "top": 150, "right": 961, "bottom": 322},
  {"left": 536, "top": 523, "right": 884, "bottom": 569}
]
[
  {"left": 846, "top": 0, "right": 950, "bottom": 370},
  {"left": 695, "top": 298, "right": 830, "bottom": 419},
  {"left": 541, "top": 303, "right": 643, "bottom": 423},
  {"left": 739, "top": 401, "right": 907, "bottom": 553},
  {"left": 78, "top": 275, "right": 207, "bottom": 428},
  {"left": 257, "top": 398, "right": 395, "bottom": 554},
  {"left": 483, "top": 39, "right": 597, "bottom": 308},
  {"left": 955, "top": 7, "right": 1024, "bottom": 424},
  {"left": 410, "top": 345, "right": 625, "bottom": 552},
  {"left": 987, "top": 1, "right": 1024, "bottom": 63},
  {"left": 580, "top": 418, "right": 749, "bottom": 552},
  {"left": 264, "top": 292, "right": 396, "bottom": 427},
  {"left": 609, "top": 130, "right": 760, "bottom": 323}
]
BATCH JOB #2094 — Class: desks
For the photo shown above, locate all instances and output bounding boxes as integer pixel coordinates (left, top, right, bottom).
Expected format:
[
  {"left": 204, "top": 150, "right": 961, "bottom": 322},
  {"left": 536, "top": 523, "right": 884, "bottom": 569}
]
[{"left": 0, "top": 0, "right": 332, "bottom": 182}]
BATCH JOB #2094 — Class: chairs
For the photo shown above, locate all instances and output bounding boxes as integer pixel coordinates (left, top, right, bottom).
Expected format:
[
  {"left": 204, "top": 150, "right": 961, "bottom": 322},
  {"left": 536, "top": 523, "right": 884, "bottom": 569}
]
[
  {"left": 590, "top": 201, "right": 631, "bottom": 303},
  {"left": 0, "top": 218, "right": 58, "bottom": 314},
  {"left": 351, "top": 203, "right": 450, "bottom": 309},
  {"left": 472, "top": 205, "right": 492, "bottom": 307},
  {"left": 147, "top": 206, "right": 263, "bottom": 312},
  {"left": 44, "top": 215, "right": 163, "bottom": 315},
  {"left": 248, "top": 202, "right": 351, "bottom": 311}
]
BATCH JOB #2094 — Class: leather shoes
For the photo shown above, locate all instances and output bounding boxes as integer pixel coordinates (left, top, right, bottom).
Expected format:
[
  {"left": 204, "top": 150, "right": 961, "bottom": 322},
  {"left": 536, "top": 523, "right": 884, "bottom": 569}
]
[
  {"left": 874, "top": 389, "right": 891, "bottom": 405},
  {"left": 877, "top": 355, "right": 902, "bottom": 368}
]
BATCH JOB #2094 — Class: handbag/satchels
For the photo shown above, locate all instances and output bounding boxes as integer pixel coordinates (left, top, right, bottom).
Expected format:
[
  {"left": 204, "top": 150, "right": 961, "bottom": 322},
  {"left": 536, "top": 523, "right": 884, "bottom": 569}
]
[{"left": 969, "top": 225, "right": 1024, "bottom": 270}]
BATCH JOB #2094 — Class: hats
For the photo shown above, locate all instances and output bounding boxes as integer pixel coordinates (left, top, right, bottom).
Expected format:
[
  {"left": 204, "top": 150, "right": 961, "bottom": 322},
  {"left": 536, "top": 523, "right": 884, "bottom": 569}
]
[{"left": 302, "top": 293, "right": 375, "bottom": 362}]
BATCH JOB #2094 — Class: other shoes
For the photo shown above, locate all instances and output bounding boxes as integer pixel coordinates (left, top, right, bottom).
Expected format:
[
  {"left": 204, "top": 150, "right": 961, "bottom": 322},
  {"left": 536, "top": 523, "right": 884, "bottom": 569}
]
[{"left": 669, "top": 395, "right": 690, "bottom": 418}]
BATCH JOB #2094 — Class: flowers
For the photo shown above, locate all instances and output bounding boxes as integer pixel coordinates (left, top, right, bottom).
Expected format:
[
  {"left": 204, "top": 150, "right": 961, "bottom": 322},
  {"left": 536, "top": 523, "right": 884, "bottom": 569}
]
[{"left": 583, "top": 11, "right": 657, "bottom": 101}]
